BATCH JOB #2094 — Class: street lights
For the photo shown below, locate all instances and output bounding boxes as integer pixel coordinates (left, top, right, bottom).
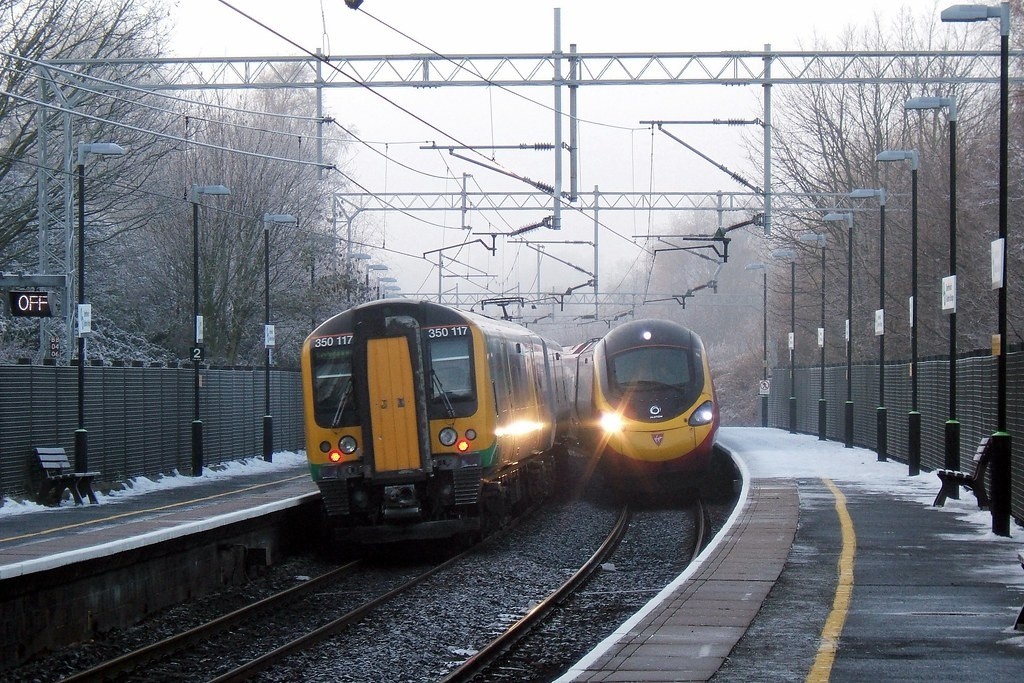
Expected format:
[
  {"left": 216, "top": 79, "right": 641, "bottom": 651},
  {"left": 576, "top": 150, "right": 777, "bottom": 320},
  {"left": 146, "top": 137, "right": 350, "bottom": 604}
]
[
  {"left": 343, "top": 251, "right": 401, "bottom": 312},
  {"left": 263, "top": 210, "right": 297, "bottom": 465},
  {"left": 904, "top": 95, "right": 960, "bottom": 500},
  {"left": 772, "top": 250, "right": 797, "bottom": 435},
  {"left": 874, "top": 149, "right": 923, "bottom": 476},
  {"left": 939, "top": 1, "right": 1012, "bottom": 536},
  {"left": 800, "top": 233, "right": 826, "bottom": 442},
  {"left": 849, "top": 189, "right": 888, "bottom": 461},
  {"left": 188, "top": 183, "right": 235, "bottom": 475},
  {"left": 744, "top": 261, "right": 769, "bottom": 428},
  {"left": 821, "top": 212, "right": 854, "bottom": 451},
  {"left": 76, "top": 140, "right": 128, "bottom": 497}
]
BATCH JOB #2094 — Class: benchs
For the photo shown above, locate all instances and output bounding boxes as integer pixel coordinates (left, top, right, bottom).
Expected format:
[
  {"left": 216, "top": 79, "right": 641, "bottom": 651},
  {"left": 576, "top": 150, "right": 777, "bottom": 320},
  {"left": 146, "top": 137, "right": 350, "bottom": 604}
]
[
  {"left": 34, "top": 447, "right": 101, "bottom": 506},
  {"left": 935, "top": 437, "right": 989, "bottom": 509}
]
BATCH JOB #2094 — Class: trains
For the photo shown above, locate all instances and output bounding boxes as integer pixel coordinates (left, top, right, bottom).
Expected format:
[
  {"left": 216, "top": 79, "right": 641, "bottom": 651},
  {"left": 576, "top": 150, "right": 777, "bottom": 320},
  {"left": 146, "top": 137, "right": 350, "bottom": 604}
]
[
  {"left": 299, "top": 296, "right": 565, "bottom": 552},
  {"left": 560, "top": 316, "right": 719, "bottom": 497}
]
